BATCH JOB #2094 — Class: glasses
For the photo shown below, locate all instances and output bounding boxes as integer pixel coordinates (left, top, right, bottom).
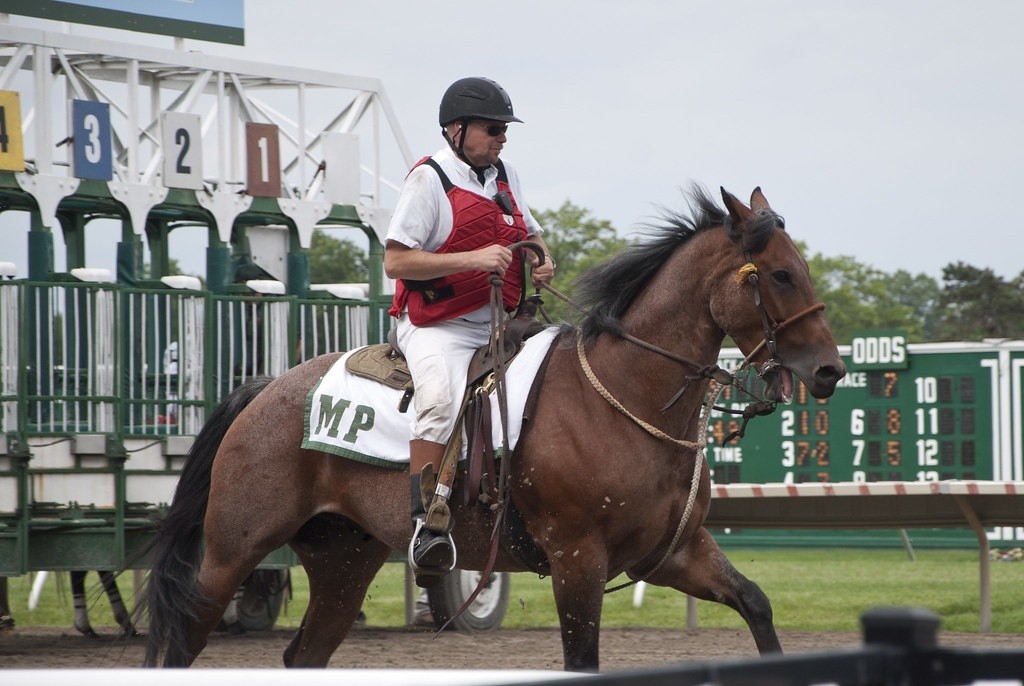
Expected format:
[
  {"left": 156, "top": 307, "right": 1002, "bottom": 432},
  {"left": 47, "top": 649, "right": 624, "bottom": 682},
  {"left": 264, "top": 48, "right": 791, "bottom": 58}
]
[{"left": 473, "top": 120, "right": 508, "bottom": 136}]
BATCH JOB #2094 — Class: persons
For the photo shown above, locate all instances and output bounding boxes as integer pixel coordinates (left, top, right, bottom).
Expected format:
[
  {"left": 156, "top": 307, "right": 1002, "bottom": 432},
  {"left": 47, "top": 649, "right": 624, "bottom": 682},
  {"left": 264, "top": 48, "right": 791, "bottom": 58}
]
[{"left": 384, "top": 75, "right": 555, "bottom": 589}]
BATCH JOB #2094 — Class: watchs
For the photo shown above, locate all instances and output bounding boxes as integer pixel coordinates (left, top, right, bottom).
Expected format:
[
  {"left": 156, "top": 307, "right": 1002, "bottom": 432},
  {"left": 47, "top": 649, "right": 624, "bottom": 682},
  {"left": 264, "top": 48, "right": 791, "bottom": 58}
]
[{"left": 545, "top": 253, "right": 557, "bottom": 271}]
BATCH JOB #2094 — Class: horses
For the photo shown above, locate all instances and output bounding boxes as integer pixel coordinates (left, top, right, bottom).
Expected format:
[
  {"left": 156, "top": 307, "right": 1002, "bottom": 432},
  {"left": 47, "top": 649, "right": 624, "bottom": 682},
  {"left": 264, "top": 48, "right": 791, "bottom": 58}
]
[{"left": 138, "top": 184, "right": 851, "bottom": 669}]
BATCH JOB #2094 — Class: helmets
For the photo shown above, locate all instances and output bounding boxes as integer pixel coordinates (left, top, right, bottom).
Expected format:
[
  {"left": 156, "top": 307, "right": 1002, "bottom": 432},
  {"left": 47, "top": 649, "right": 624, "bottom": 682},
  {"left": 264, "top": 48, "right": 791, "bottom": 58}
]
[{"left": 438, "top": 78, "right": 524, "bottom": 125}]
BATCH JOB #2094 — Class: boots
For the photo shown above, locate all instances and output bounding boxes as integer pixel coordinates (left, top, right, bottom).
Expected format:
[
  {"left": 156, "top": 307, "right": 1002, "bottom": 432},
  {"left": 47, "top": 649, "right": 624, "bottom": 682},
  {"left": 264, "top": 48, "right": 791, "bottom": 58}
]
[{"left": 409, "top": 472, "right": 453, "bottom": 588}]
